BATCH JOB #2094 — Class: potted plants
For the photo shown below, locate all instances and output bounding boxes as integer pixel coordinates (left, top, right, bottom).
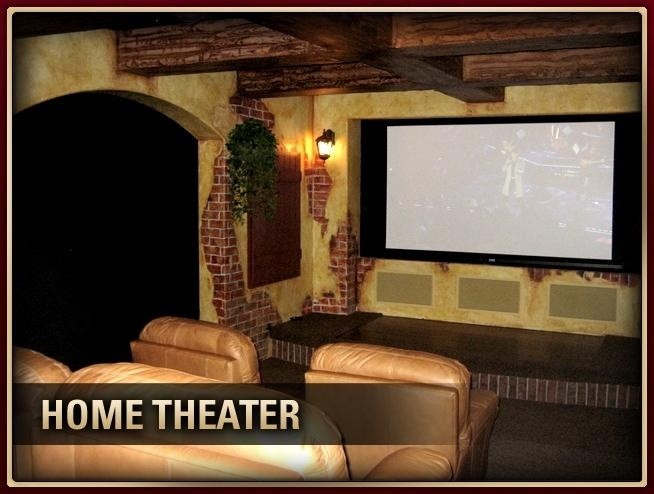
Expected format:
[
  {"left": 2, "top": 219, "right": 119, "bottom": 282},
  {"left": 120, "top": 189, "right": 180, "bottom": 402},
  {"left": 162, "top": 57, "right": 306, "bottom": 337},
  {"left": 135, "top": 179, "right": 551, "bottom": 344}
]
[{"left": 224, "top": 118, "right": 279, "bottom": 227}]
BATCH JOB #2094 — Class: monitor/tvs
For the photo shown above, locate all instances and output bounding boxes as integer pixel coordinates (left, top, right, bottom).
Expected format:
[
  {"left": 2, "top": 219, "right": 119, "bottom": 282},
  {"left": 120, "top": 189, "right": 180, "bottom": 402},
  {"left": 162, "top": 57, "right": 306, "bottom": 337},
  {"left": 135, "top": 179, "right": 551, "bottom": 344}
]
[{"left": 359, "top": 112, "right": 641, "bottom": 274}]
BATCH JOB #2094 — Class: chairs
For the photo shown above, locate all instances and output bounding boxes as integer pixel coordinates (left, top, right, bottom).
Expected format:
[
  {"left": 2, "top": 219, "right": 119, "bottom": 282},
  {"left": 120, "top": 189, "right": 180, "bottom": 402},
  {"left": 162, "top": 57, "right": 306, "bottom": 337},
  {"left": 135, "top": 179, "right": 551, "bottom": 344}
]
[{"left": 11, "top": 314, "right": 508, "bottom": 483}]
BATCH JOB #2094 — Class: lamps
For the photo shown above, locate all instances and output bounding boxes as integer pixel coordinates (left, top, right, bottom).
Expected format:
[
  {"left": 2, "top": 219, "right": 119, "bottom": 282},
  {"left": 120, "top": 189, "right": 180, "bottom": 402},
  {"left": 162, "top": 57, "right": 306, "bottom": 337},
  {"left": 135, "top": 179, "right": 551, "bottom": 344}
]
[{"left": 315, "top": 128, "right": 335, "bottom": 165}]
